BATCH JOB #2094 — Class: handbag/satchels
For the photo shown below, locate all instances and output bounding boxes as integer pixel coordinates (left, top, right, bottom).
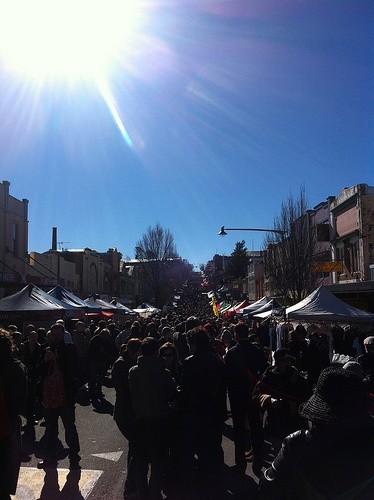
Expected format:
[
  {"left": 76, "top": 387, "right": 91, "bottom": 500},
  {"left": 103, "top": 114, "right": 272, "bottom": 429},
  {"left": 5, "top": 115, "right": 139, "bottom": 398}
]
[{"left": 42, "top": 375, "right": 68, "bottom": 409}]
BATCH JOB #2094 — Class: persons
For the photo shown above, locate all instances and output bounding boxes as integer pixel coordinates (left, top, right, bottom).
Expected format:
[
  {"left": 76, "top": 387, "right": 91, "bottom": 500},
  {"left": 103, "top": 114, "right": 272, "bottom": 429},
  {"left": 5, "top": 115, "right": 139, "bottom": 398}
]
[{"left": 0, "top": 284, "right": 373, "bottom": 500}]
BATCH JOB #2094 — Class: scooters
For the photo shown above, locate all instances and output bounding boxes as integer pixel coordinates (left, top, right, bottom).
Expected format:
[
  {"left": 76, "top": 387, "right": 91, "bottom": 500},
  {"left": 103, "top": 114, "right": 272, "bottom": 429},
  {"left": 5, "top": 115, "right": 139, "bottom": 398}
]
[{"left": 261, "top": 395, "right": 299, "bottom": 468}]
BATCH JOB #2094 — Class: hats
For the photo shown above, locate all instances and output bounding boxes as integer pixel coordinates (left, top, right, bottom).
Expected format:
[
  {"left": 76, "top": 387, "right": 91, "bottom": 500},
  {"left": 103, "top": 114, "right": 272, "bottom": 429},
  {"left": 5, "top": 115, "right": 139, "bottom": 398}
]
[
  {"left": 274, "top": 349, "right": 295, "bottom": 365},
  {"left": 342, "top": 361, "right": 363, "bottom": 376},
  {"left": 299, "top": 366, "right": 373, "bottom": 425}
]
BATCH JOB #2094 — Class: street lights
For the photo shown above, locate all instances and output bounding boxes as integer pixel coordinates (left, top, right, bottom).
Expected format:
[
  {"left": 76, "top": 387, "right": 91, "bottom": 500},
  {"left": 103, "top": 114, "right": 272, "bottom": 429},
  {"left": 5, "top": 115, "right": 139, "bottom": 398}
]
[{"left": 219, "top": 225, "right": 286, "bottom": 258}]
